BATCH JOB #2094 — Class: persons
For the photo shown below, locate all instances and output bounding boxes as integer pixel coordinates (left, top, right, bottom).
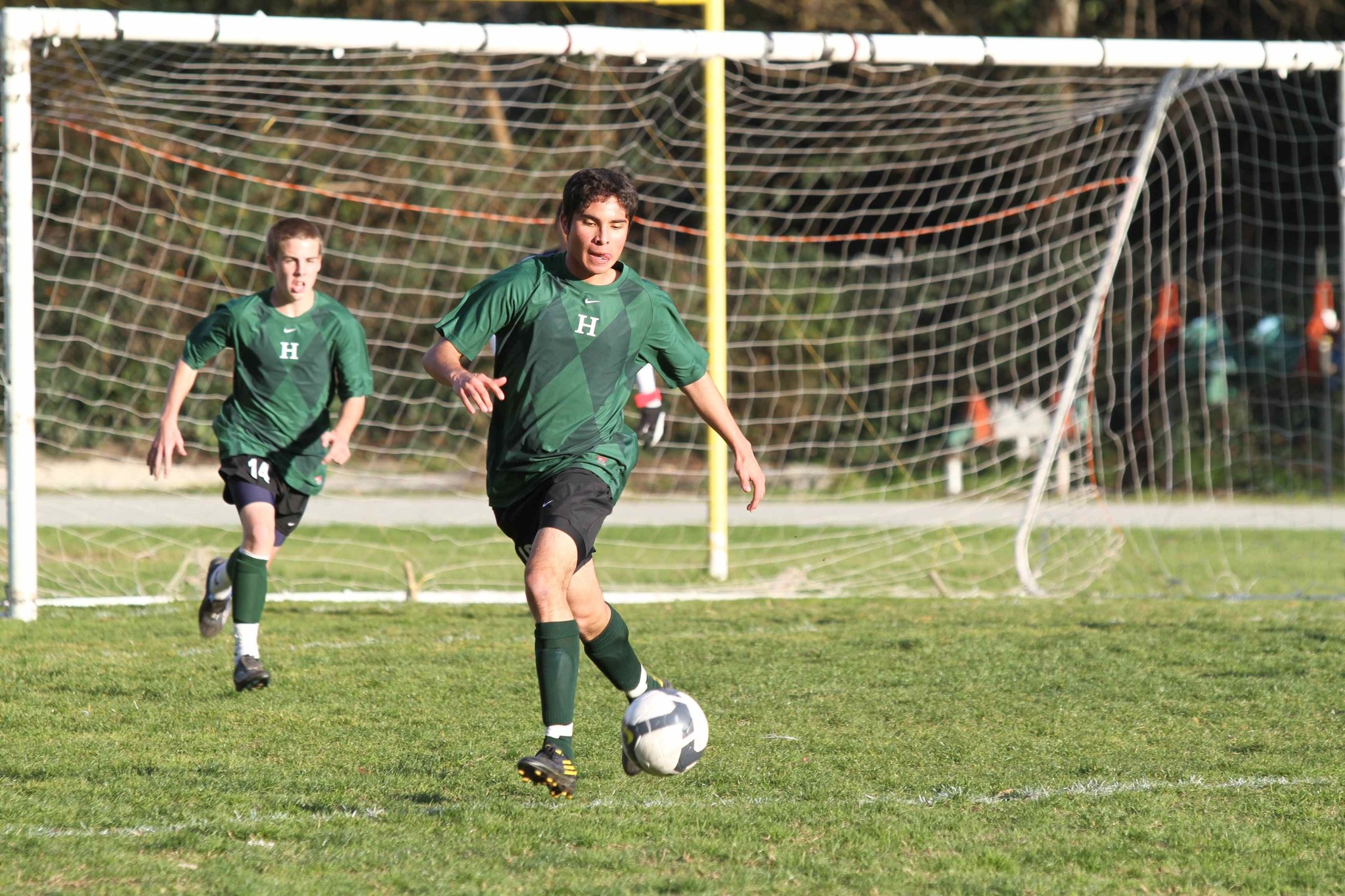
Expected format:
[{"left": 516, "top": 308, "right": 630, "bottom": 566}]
[
  {"left": 491, "top": 333, "right": 667, "bottom": 452},
  {"left": 147, "top": 216, "right": 375, "bottom": 692},
  {"left": 421, "top": 167, "right": 765, "bottom": 799}
]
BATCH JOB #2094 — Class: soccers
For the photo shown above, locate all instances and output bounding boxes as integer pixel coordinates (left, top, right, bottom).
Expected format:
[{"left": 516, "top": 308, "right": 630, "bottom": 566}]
[{"left": 621, "top": 689, "right": 709, "bottom": 777}]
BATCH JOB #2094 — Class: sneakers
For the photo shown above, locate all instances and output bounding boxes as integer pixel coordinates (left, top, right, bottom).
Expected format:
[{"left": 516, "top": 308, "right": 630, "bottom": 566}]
[
  {"left": 233, "top": 655, "right": 269, "bottom": 691},
  {"left": 517, "top": 743, "right": 577, "bottom": 799},
  {"left": 622, "top": 671, "right": 671, "bottom": 776},
  {"left": 199, "top": 558, "right": 234, "bottom": 638}
]
